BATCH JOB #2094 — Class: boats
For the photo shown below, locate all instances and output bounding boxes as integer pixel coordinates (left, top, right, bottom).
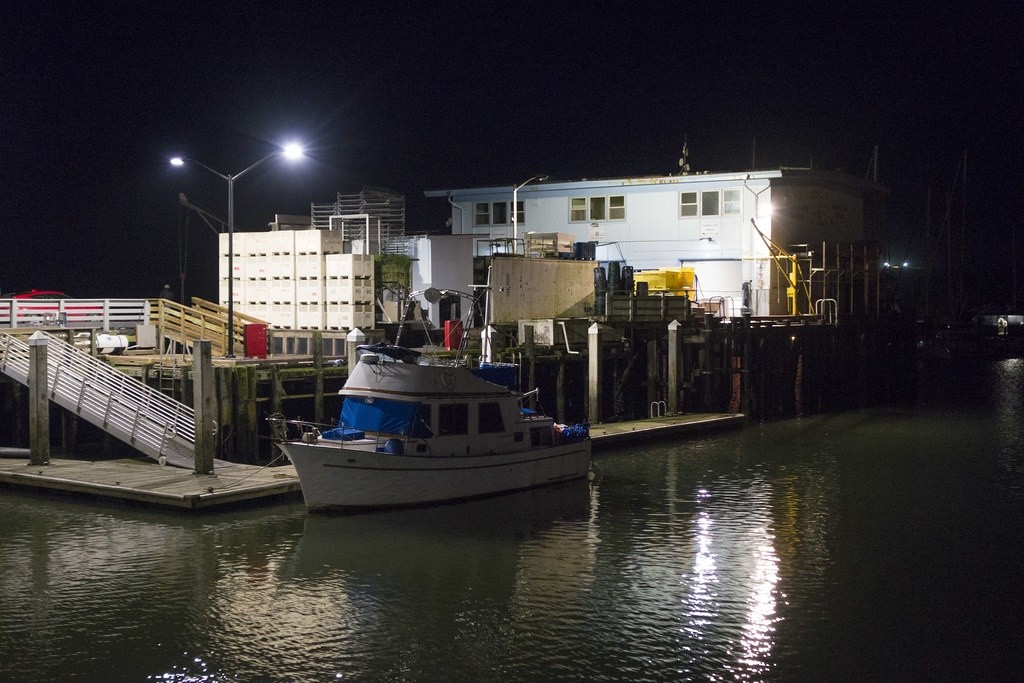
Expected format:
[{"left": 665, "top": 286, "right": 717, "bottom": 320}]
[{"left": 265, "top": 265, "right": 592, "bottom": 514}]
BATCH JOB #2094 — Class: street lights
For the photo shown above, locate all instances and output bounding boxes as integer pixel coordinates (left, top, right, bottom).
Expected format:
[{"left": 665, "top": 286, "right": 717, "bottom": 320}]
[
  {"left": 170, "top": 143, "right": 305, "bottom": 359},
  {"left": 513, "top": 175, "right": 549, "bottom": 253}
]
